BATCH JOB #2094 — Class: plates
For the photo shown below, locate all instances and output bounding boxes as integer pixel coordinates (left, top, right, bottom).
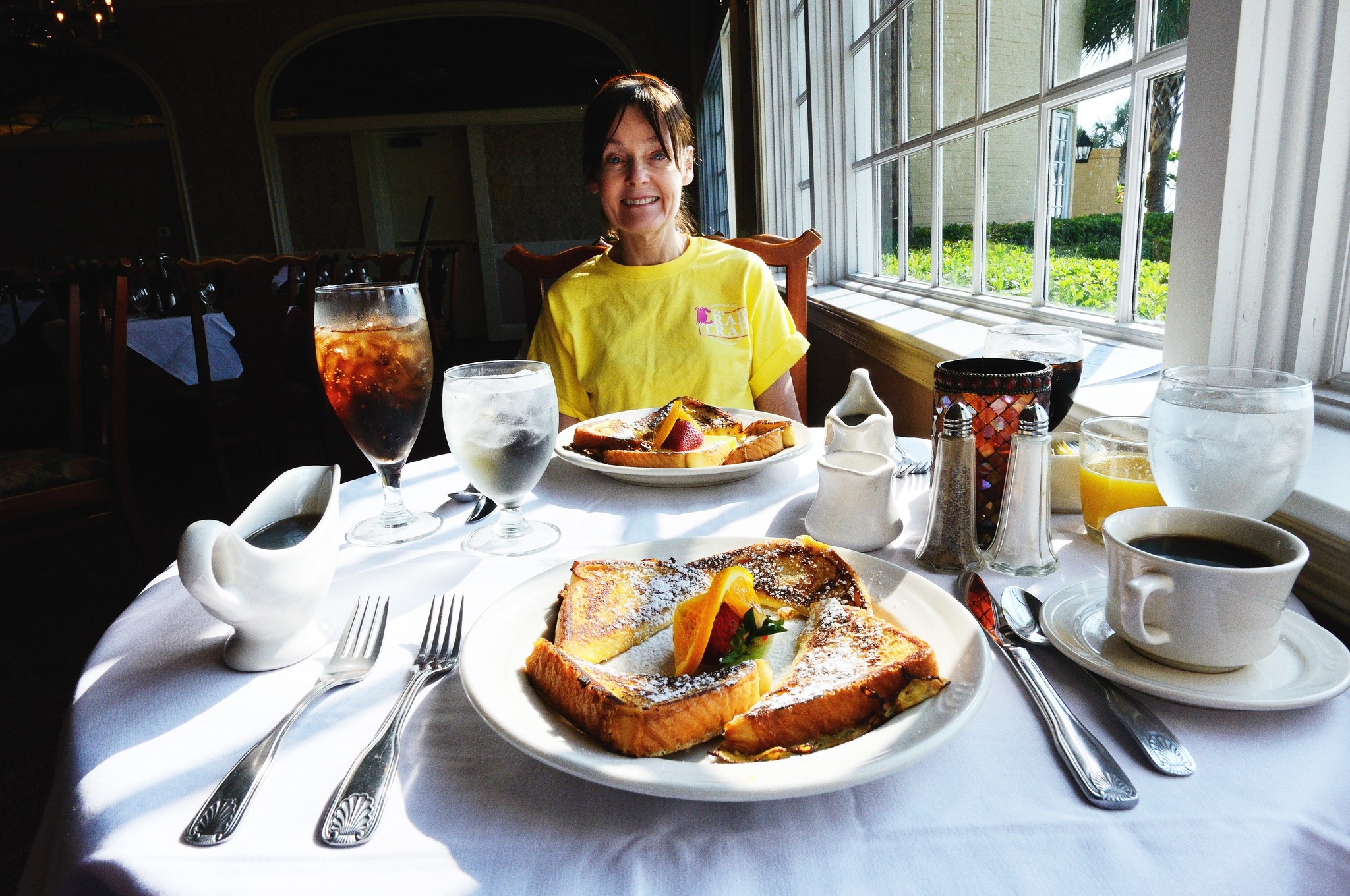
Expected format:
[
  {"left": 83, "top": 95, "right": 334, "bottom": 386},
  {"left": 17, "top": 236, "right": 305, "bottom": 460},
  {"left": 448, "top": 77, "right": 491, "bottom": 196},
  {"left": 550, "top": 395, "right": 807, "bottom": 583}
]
[
  {"left": 554, "top": 405, "right": 812, "bottom": 488},
  {"left": 461, "top": 532, "right": 992, "bottom": 803},
  {"left": 1040, "top": 577, "right": 1350, "bottom": 711}
]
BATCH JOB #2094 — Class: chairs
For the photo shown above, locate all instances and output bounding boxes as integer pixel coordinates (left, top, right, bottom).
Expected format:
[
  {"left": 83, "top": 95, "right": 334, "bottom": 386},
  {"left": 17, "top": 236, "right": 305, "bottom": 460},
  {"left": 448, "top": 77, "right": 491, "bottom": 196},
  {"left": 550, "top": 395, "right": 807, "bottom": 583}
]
[
  {"left": 498, "top": 226, "right": 828, "bottom": 422},
  {"left": 0, "top": 250, "right": 463, "bottom": 734}
]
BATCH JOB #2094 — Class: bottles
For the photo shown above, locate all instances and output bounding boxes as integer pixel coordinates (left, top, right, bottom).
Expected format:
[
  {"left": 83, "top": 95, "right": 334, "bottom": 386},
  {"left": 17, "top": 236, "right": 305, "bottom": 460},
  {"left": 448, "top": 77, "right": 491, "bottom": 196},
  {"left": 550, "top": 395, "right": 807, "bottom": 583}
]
[
  {"left": 917, "top": 404, "right": 988, "bottom": 572},
  {"left": 981, "top": 403, "right": 1060, "bottom": 575}
]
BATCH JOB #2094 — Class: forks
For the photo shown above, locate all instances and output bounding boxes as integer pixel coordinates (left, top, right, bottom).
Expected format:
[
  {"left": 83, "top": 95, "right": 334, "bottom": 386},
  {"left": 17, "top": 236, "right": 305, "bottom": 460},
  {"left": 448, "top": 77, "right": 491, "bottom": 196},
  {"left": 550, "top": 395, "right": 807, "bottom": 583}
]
[
  {"left": 321, "top": 590, "right": 466, "bottom": 851},
  {"left": 180, "top": 595, "right": 391, "bottom": 846},
  {"left": 893, "top": 435, "right": 933, "bottom": 479}
]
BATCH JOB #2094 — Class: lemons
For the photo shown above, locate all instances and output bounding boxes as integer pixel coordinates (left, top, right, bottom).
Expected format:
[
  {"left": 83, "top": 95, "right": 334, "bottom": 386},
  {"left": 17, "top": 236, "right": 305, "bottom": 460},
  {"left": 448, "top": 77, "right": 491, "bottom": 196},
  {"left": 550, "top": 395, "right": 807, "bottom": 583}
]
[{"left": 666, "top": 562, "right": 760, "bottom": 680}]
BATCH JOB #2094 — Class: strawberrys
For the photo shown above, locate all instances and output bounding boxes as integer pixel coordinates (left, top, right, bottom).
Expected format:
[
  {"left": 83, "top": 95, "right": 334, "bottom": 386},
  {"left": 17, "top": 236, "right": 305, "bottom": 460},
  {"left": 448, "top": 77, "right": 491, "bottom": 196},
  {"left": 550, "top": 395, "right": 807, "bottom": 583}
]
[{"left": 708, "top": 601, "right": 770, "bottom": 665}]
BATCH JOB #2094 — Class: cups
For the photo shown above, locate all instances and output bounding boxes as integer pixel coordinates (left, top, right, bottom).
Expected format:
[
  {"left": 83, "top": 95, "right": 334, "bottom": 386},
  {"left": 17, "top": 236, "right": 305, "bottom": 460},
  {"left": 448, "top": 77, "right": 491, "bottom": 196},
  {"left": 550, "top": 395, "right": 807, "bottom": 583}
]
[
  {"left": 805, "top": 450, "right": 904, "bottom": 553},
  {"left": 179, "top": 464, "right": 341, "bottom": 672},
  {"left": 1101, "top": 507, "right": 1310, "bottom": 673},
  {"left": 1078, "top": 414, "right": 1169, "bottom": 545},
  {"left": 1147, "top": 365, "right": 1315, "bottom": 522},
  {"left": 823, "top": 368, "right": 896, "bottom": 468}
]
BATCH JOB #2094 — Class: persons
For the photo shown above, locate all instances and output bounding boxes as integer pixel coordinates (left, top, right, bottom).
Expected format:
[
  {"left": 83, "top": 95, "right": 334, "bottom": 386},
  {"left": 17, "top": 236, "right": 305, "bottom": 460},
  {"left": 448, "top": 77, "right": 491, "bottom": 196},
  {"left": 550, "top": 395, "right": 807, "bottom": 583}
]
[{"left": 522, "top": 72, "right": 811, "bottom": 435}]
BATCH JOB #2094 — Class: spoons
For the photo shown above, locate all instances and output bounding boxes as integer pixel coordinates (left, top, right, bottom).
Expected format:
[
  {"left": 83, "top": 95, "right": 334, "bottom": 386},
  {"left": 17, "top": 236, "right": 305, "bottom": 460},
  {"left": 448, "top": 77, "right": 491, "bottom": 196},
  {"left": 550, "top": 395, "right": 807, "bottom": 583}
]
[
  {"left": 448, "top": 483, "right": 482, "bottom": 502},
  {"left": 1000, "top": 585, "right": 1197, "bottom": 779}
]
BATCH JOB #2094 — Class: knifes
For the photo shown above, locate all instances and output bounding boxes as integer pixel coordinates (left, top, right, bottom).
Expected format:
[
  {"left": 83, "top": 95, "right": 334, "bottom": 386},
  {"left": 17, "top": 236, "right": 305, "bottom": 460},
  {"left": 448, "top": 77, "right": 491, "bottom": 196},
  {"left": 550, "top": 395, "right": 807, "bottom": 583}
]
[
  {"left": 466, "top": 495, "right": 496, "bottom": 522},
  {"left": 958, "top": 572, "right": 1138, "bottom": 812}
]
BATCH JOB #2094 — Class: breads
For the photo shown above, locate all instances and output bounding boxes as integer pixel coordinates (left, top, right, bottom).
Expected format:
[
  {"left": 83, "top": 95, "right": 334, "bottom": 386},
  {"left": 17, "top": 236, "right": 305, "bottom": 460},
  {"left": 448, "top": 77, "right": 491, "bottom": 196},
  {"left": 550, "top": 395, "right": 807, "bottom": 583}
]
[
  {"left": 677, "top": 532, "right": 873, "bottom": 626},
  {"left": 721, "top": 594, "right": 950, "bottom": 754},
  {"left": 552, "top": 553, "right": 715, "bottom": 671},
  {"left": 524, "top": 632, "right": 773, "bottom": 760},
  {"left": 569, "top": 396, "right": 797, "bottom": 474}
]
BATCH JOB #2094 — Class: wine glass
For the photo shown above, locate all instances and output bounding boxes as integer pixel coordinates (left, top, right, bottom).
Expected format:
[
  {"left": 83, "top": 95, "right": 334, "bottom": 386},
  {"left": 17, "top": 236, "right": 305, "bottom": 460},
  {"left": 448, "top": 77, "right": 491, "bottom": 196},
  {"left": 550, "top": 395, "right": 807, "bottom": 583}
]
[
  {"left": 314, "top": 280, "right": 445, "bottom": 545},
  {"left": 129, "top": 287, "right": 151, "bottom": 319},
  {"left": 201, "top": 283, "right": 218, "bottom": 313},
  {"left": 441, "top": 361, "right": 565, "bottom": 555}
]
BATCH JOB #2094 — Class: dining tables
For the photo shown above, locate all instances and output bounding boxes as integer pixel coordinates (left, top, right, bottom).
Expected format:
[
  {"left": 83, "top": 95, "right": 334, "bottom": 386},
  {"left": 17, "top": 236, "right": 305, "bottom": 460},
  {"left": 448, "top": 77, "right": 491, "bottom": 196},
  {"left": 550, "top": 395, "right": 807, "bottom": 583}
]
[{"left": 51, "top": 434, "right": 1350, "bottom": 891}]
